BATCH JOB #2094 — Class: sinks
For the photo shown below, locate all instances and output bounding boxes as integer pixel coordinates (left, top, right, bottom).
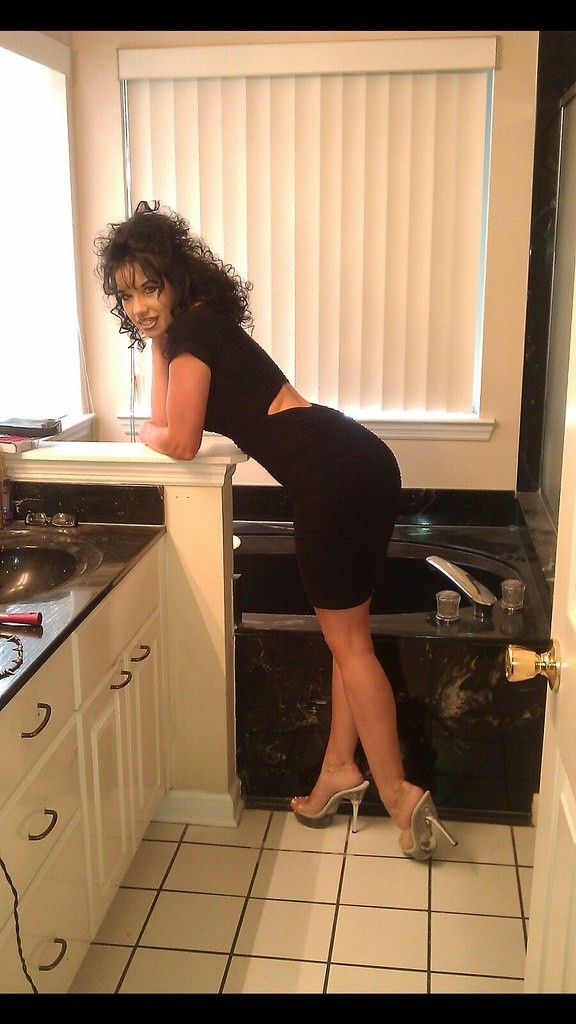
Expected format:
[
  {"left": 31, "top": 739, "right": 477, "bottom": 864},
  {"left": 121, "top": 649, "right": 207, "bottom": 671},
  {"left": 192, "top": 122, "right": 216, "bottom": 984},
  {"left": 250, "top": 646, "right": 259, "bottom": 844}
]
[{"left": 0, "top": 545, "right": 78, "bottom": 605}]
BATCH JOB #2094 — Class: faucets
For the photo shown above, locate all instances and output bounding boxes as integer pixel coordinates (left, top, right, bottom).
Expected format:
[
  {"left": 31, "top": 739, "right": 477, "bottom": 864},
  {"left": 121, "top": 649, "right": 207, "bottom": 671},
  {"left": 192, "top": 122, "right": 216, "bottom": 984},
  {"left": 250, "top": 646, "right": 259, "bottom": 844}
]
[{"left": 424, "top": 554, "right": 497, "bottom": 619}]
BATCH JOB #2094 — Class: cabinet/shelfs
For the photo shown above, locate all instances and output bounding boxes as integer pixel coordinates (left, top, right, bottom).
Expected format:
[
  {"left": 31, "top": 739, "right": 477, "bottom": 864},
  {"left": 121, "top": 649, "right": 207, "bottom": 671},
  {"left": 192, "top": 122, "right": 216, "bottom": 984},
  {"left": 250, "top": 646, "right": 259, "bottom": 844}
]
[
  {"left": 0, "top": 632, "right": 91, "bottom": 992},
  {"left": 70, "top": 544, "right": 164, "bottom": 944}
]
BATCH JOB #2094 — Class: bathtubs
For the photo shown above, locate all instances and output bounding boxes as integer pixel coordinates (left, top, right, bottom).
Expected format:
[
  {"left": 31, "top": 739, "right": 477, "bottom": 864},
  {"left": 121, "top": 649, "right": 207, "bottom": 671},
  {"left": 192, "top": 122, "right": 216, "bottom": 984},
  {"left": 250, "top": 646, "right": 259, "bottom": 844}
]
[{"left": 234, "top": 522, "right": 550, "bottom": 827}]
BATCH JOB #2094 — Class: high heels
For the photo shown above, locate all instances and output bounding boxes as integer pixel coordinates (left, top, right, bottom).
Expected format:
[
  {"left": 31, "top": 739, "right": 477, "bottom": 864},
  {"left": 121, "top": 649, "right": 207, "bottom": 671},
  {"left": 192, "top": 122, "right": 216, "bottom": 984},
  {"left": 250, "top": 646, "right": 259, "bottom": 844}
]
[
  {"left": 397, "top": 790, "right": 459, "bottom": 862},
  {"left": 289, "top": 780, "right": 370, "bottom": 834}
]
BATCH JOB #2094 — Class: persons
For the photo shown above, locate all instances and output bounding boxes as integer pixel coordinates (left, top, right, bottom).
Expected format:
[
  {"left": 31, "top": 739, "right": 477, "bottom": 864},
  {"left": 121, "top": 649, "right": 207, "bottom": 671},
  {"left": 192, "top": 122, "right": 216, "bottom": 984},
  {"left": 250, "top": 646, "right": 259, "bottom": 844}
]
[{"left": 93, "top": 202, "right": 459, "bottom": 861}]
